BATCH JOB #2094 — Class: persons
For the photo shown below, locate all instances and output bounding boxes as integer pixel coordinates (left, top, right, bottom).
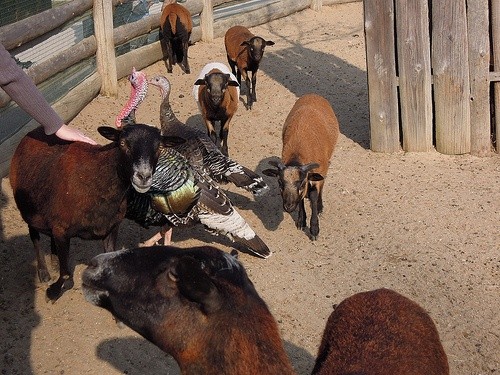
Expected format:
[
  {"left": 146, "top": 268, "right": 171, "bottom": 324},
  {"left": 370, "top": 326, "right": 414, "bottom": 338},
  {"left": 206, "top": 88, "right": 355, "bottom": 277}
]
[{"left": 0, "top": 41, "right": 97, "bottom": 146}]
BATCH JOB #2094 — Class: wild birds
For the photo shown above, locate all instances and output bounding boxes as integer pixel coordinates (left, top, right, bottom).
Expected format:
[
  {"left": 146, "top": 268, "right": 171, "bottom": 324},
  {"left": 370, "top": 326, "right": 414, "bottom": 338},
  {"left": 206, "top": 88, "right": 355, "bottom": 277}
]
[{"left": 115, "top": 67, "right": 271, "bottom": 259}]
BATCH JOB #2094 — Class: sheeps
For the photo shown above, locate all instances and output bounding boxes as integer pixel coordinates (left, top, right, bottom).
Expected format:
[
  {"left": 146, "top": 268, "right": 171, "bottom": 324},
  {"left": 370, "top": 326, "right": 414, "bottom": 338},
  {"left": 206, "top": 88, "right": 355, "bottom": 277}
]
[
  {"left": 159, "top": 0, "right": 192, "bottom": 74},
  {"left": 8, "top": 124, "right": 187, "bottom": 304},
  {"left": 192, "top": 62, "right": 241, "bottom": 157},
  {"left": 81, "top": 246, "right": 449, "bottom": 375},
  {"left": 225, "top": 25, "right": 275, "bottom": 110},
  {"left": 262, "top": 93, "right": 339, "bottom": 241}
]
